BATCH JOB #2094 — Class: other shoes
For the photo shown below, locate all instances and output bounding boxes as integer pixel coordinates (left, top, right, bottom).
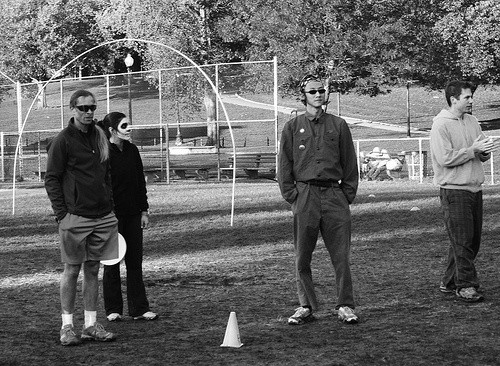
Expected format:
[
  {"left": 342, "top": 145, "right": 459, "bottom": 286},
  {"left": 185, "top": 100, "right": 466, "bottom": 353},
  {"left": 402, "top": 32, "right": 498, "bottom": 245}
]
[
  {"left": 456, "top": 287, "right": 484, "bottom": 304},
  {"left": 439, "top": 281, "right": 456, "bottom": 293}
]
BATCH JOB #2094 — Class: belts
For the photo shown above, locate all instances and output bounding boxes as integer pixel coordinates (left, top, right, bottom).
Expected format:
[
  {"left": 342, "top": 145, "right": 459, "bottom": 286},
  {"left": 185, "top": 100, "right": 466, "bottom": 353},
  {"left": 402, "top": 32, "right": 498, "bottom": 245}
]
[{"left": 300, "top": 179, "right": 339, "bottom": 187}]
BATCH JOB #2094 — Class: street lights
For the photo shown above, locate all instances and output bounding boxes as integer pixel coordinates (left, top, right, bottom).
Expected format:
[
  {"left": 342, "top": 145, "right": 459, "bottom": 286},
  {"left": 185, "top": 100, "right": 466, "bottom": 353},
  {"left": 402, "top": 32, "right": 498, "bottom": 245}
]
[{"left": 124, "top": 54, "right": 135, "bottom": 126}]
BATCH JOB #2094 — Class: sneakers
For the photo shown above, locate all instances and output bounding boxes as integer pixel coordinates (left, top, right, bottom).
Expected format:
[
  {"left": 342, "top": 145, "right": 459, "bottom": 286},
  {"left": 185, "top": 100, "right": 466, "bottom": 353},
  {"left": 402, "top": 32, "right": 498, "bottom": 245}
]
[
  {"left": 106, "top": 313, "right": 122, "bottom": 321},
  {"left": 59, "top": 324, "right": 79, "bottom": 346},
  {"left": 287, "top": 306, "right": 313, "bottom": 324},
  {"left": 337, "top": 305, "right": 358, "bottom": 324},
  {"left": 81, "top": 321, "right": 116, "bottom": 341},
  {"left": 133, "top": 311, "right": 158, "bottom": 321}
]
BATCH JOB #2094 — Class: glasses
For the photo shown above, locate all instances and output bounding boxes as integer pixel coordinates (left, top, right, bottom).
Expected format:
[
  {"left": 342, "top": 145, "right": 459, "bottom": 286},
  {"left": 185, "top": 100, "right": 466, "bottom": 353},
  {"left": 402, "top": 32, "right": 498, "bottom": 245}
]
[
  {"left": 76, "top": 104, "right": 97, "bottom": 112},
  {"left": 305, "top": 89, "right": 326, "bottom": 95}
]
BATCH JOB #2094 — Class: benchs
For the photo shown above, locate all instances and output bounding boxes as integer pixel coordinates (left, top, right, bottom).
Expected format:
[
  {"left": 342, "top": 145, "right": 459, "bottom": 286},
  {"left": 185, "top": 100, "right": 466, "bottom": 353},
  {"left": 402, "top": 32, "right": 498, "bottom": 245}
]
[
  {"left": 219, "top": 151, "right": 275, "bottom": 180},
  {"left": 142, "top": 153, "right": 219, "bottom": 182}
]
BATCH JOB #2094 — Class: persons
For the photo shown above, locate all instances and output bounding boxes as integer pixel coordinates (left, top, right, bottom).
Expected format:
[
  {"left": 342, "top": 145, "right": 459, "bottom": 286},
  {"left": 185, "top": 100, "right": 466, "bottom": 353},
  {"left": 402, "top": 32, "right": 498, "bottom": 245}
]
[
  {"left": 97, "top": 112, "right": 159, "bottom": 322},
  {"left": 45, "top": 89, "right": 118, "bottom": 346},
  {"left": 277, "top": 74, "right": 359, "bottom": 325},
  {"left": 429, "top": 80, "right": 500, "bottom": 303}
]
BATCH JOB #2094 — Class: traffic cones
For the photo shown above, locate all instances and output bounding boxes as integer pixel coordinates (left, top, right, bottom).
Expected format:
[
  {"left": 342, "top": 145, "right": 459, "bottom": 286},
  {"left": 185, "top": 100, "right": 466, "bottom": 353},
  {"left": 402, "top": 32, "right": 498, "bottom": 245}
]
[{"left": 220, "top": 311, "right": 245, "bottom": 348}]
